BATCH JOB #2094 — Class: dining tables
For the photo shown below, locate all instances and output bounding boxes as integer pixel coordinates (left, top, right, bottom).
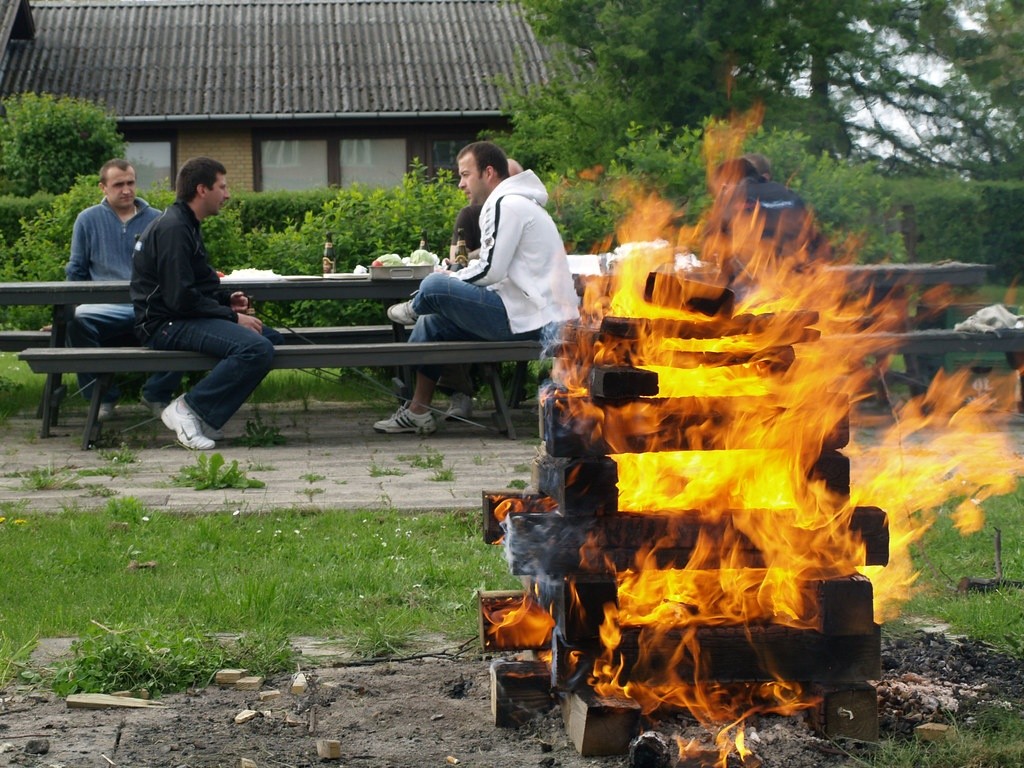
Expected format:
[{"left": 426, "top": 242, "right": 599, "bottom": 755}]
[{"left": 0, "top": 263, "right": 984, "bottom": 435}]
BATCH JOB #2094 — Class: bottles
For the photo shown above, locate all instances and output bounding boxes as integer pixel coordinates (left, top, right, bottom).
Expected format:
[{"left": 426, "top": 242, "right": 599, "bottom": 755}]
[
  {"left": 419, "top": 229, "right": 430, "bottom": 251},
  {"left": 454, "top": 228, "right": 468, "bottom": 268},
  {"left": 322, "top": 231, "right": 336, "bottom": 273}
]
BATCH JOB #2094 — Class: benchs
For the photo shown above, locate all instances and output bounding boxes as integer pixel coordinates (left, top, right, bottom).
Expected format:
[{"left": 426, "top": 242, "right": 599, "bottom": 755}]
[
  {"left": 0, "top": 324, "right": 413, "bottom": 419},
  {"left": 19, "top": 326, "right": 1024, "bottom": 451}
]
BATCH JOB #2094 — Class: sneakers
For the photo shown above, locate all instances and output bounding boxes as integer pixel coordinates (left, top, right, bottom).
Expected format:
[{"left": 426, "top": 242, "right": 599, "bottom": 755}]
[
  {"left": 160, "top": 401, "right": 216, "bottom": 450},
  {"left": 443, "top": 391, "right": 474, "bottom": 422},
  {"left": 372, "top": 401, "right": 438, "bottom": 435},
  {"left": 386, "top": 299, "right": 423, "bottom": 326},
  {"left": 170, "top": 392, "right": 225, "bottom": 441}
]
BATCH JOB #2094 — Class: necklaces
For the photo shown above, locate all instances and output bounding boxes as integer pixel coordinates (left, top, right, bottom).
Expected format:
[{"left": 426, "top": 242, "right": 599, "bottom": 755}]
[{"left": 134, "top": 206, "right": 138, "bottom": 215}]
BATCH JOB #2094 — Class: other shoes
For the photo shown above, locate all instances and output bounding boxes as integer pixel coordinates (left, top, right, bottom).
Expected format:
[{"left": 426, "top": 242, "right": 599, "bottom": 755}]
[
  {"left": 97, "top": 393, "right": 121, "bottom": 421},
  {"left": 139, "top": 394, "right": 168, "bottom": 420}
]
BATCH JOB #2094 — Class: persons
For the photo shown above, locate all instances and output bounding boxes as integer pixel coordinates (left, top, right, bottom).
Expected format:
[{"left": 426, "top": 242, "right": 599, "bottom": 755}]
[
  {"left": 40, "top": 158, "right": 182, "bottom": 421},
  {"left": 373, "top": 142, "right": 581, "bottom": 435},
  {"left": 742, "top": 153, "right": 772, "bottom": 184},
  {"left": 716, "top": 159, "right": 818, "bottom": 265},
  {"left": 131, "top": 157, "right": 286, "bottom": 450}
]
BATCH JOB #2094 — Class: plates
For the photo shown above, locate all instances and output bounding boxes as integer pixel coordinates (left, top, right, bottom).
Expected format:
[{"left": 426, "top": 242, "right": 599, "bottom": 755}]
[
  {"left": 324, "top": 273, "right": 369, "bottom": 278},
  {"left": 282, "top": 275, "right": 323, "bottom": 281}
]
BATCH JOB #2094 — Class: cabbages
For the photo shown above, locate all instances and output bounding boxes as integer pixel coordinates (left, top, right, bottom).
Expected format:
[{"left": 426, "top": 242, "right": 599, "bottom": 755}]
[{"left": 377, "top": 250, "right": 442, "bottom": 267}]
